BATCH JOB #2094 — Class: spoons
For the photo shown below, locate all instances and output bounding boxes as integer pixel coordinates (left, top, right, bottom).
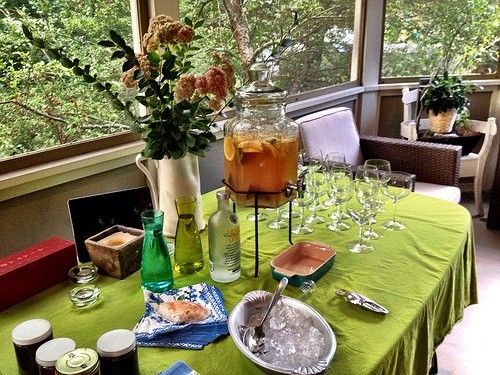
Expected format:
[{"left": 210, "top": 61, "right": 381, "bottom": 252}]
[{"left": 243, "top": 277, "right": 289, "bottom": 352}]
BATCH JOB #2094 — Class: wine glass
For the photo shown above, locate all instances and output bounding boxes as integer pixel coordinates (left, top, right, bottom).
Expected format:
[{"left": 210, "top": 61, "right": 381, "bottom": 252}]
[{"left": 247, "top": 148, "right": 412, "bottom": 253}]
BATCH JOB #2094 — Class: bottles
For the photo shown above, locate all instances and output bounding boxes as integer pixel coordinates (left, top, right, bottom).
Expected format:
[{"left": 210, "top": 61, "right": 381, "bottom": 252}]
[
  {"left": 208, "top": 190, "right": 243, "bottom": 284},
  {"left": 223, "top": 62, "right": 301, "bottom": 209},
  {"left": 174, "top": 195, "right": 204, "bottom": 275},
  {"left": 95, "top": 329, "right": 141, "bottom": 375},
  {"left": 11, "top": 318, "right": 102, "bottom": 375},
  {"left": 140, "top": 210, "right": 174, "bottom": 293}
]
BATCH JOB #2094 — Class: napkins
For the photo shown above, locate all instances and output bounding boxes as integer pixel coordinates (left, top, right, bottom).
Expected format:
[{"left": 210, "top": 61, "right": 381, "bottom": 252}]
[
  {"left": 131, "top": 283, "right": 229, "bottom": 350},
  {"left": 158, "top": 361, "right": 201, "bottom": 375}
]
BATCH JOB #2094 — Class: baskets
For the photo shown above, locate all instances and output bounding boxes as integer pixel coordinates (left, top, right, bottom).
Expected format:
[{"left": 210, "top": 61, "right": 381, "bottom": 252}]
[{"left": 429, "top": 107, "right": 458, "bottom": 132}]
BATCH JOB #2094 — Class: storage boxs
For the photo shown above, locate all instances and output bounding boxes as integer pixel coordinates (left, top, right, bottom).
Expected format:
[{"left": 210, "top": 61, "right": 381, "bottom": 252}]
[
  {"left": 85, "top": 225, "right": 144, "bottom": 280},
  {"left": 0, "top": 238, "right": 79, "bottom": 312}
]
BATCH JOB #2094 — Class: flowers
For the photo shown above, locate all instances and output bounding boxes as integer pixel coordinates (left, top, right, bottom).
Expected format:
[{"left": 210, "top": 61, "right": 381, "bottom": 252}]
[{"left": 22, "top": 0, "right": 348, "bottom": 160}]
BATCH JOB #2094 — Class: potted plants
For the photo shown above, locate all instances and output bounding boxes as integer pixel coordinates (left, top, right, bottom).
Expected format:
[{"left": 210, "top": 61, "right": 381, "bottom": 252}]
[{"left": 423, "top": 70, "right": 476, "bottom": 133}]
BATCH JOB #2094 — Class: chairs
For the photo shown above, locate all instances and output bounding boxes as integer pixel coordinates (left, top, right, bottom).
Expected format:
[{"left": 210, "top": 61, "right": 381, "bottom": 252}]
[{"left": 295, "top": 107, "right": 462, "bottom": 204}]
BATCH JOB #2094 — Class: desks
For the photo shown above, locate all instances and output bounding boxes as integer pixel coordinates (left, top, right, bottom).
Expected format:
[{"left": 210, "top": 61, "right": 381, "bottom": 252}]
[{"left": 0, "top": 169, "right": 479, "bottom": 375}]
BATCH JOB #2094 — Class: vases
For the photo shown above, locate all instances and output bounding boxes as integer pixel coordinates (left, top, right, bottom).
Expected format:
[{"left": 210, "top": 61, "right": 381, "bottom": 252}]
[{"left": 135, "top": 151, "right": 206, "bottom": 238}]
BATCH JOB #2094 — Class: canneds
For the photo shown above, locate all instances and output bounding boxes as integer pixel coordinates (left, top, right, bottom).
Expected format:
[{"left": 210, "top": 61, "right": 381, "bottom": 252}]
[{"left": 11, "top": 319, "right": 139, "bottom": 375}]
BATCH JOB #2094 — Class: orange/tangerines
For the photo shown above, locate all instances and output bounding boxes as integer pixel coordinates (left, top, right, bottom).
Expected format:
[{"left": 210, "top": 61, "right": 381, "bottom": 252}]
[{"left": 224, "top": 134, "right": 297, "bottom": 162}]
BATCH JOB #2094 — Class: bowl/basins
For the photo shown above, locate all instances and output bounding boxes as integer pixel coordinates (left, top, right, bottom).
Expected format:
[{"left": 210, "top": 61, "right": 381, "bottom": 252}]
[
  {"left": 227, "top": 290, "right": 338, "bottom": 375},
  {"left": 270, "top": 242, "right": 337, "bottom": 287}
]
